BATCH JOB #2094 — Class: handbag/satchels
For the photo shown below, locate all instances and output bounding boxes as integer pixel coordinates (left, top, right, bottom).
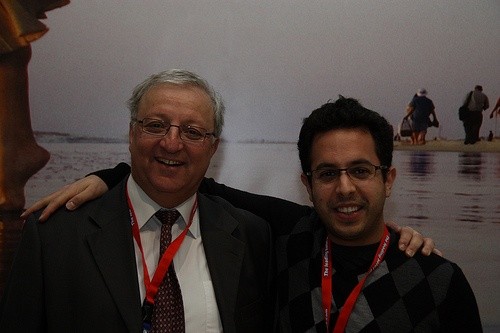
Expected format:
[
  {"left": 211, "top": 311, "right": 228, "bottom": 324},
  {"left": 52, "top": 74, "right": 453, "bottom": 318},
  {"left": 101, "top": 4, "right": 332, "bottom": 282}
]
[
  {"left": 399, "top": 118, "right": 413, "bottom": 137},
  {"left": 458, "top": 105, "right": 469, "bottom": 121}
]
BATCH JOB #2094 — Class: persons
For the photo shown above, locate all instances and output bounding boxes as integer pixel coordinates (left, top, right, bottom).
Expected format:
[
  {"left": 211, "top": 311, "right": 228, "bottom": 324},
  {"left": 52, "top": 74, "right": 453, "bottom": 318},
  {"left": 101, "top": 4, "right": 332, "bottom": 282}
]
[
  {"left": 0, "top": 69, "right": 441, "bottom": 333},
  {"left": 407, "top": 88, "right": 439, "bottom": 144},
  {"left": 460, "top": 84, "right": 490, "bottom": 145},
  {"left": 489, "top": 97, "right": 500, "bottom": 119},
  {"left": 20, "top": 94, "right": 484, "bottom": 333}
]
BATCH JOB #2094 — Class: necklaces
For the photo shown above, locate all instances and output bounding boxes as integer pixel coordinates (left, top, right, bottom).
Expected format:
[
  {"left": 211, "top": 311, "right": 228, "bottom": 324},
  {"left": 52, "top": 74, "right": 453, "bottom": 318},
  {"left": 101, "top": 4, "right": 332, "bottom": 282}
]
[
  {"left": 321, "top": 224, "right": 390, "bottom": 333},
  {"left": 122, "top": 185, "right": 198, "bottom": 333}
]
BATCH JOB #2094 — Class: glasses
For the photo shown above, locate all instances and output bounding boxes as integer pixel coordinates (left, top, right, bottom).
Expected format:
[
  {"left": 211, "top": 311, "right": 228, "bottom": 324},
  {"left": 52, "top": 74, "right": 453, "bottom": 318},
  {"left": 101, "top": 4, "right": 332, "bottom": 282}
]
[
  {"left": 306, "top": 163, "right": 387, "bottom": 182},
  {"left": 130, "top": 117, "right": 214, "bottom": 145}
]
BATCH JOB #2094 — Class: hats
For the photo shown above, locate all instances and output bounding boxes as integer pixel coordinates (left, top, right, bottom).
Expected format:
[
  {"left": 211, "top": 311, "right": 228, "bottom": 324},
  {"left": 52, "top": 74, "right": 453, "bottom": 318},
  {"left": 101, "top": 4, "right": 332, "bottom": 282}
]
[{"left": 417, "top": 88, "right": 429, "bottom": 97}]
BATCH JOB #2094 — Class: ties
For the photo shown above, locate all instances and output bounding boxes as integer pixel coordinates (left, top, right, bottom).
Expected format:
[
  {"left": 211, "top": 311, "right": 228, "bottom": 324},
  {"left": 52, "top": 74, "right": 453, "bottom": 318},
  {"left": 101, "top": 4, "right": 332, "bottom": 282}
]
[{"left": 146, "top": 210, "right": 188, "bottom": 333}]
